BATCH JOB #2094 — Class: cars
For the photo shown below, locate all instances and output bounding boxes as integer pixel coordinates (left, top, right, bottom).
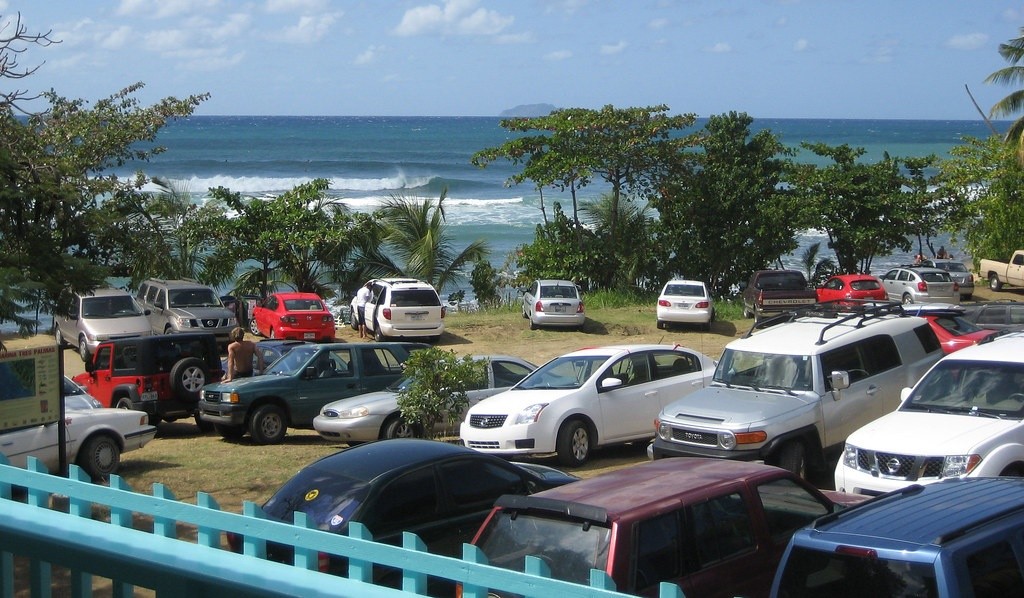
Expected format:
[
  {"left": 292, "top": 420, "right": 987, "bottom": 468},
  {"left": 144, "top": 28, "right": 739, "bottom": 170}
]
[
  {"left": 250, "top": 292, "right": 336, "bottom": 342},
  {"left": 520, "top": 278, "right": 585, "bottom": 330},
  {"left": 0, "top": 374, "right": 156, "bottom": 479},
  {"left": 656, "top": 279, "right": 715, "bottom": 331},
  {"left": 313, "top": 343, "right": 736, "bottom": 468},
  {"left": 816, "top": 275, "right": 890, "bottom": 307},
  {"left": 225, "top": 438, "right": 582, "bottom": 598},
  {"left": 219, "top": 339, "right": 348, "bottom": 380},
  {"left": 922, "top": 259, "right": 974, "bottom": 298}
]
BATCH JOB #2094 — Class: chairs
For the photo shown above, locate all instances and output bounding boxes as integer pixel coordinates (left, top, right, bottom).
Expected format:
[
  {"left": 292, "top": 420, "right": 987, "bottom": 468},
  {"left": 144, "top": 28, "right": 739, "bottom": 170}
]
[{"left": 669, "top": 358, "right": 688, "bottom": 375}]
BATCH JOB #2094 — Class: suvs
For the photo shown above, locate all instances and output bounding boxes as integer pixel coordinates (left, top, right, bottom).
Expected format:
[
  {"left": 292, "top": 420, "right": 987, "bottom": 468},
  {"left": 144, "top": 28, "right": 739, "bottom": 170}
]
[
  {"left": 348, "top": 278, "right": 446, "bottom": 342},
  {"left": 455, "top": 454, "right": 874, "bottom": 598},
  {"left": 70, "top": 332, "right": 225, "bottom": 433},
  {"left": 879, "top": 263, "right": 961, "bottom": 307},
  {"left": 771, "top": 478, "right": 1024, "bottom": 598},
  {"left": 646, "top": 298, "right": 1024, "bottom": 499},
  {"left": 136, "top": 277, "right": 240, "bottom": 349}
]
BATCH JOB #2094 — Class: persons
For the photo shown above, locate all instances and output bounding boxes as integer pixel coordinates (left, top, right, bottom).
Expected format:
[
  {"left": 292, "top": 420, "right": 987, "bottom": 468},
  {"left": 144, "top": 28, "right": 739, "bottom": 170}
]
[
  {"left": 914, "top": 254, "right": 925, "bottom": 264},
  {"left": 937, "top": 246, "right": 947, "bottom": 259},
  {"left": 221, "top": 327, "right": 263, "bottom": 384},
  {"left": 356, "top": 282, "right": 371, "bottom": 338}
]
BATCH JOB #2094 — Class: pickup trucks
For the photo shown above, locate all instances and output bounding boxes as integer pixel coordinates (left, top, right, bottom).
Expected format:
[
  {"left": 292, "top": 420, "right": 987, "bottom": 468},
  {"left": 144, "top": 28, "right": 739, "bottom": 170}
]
[
  {"left": 739, "top": 270, "right": 818, "bottom": 322},
  {"left": 980, "top": 250, "right": 1024, "bottom": 291},
  {"left": 198, "top": 342, "right": 436, "bottom": 444}
]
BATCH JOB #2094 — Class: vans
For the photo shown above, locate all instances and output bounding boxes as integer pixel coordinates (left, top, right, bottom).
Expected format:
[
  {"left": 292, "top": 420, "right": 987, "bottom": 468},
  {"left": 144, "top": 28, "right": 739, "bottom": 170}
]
[{"left": 53, "top": 283, "right": 154, "bottom": 361}]
